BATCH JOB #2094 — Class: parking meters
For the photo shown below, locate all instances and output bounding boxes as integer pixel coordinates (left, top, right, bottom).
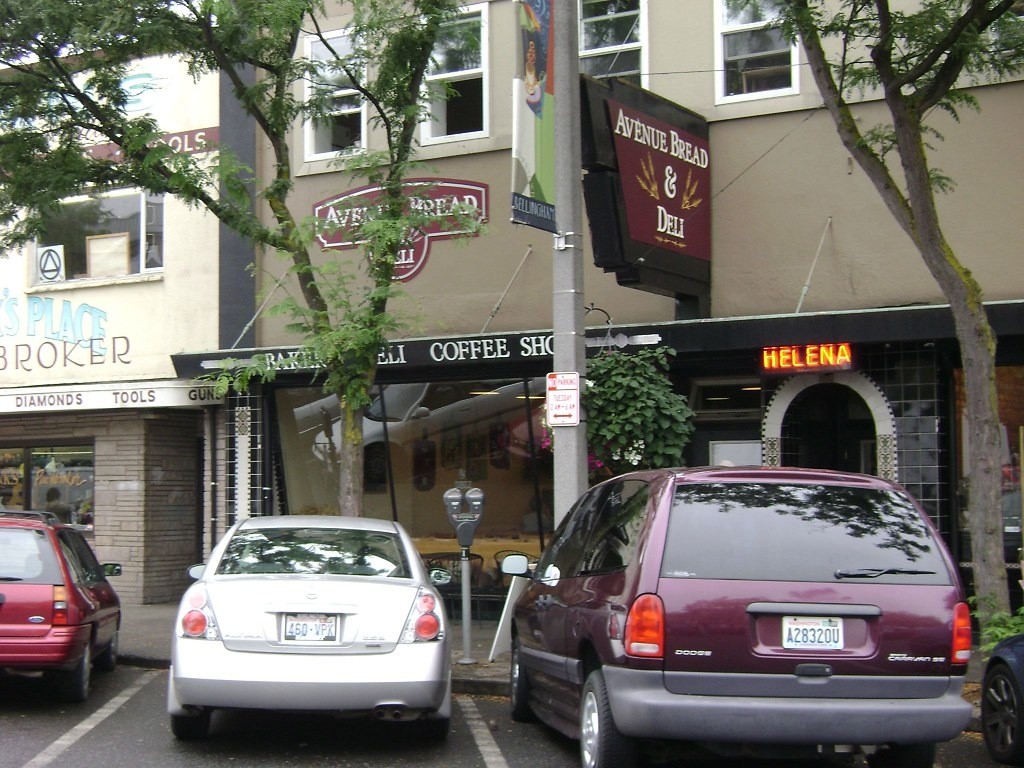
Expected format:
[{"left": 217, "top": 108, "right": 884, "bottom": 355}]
[{"left": 443, "top": 487, "right": 484, "bottom": 664}]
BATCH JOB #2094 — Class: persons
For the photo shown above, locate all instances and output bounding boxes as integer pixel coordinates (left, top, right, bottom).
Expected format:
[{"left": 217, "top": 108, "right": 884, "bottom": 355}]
[{"left": 46, "top": 488, "right": 73, "bottom": 523}]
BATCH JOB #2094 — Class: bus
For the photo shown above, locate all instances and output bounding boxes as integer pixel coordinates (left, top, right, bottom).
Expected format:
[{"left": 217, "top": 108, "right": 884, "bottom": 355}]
[{"left": 19, "top": 452, "right": 95, "bottom": 483}]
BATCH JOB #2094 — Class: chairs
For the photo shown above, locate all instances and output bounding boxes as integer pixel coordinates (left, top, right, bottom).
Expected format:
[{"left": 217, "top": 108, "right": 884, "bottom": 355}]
[
  {"left": 492, "top": 550, "right": 541, "bottom": 625},
  {"left": 429, "top": 554, "right": 484, "bottom": 623}
]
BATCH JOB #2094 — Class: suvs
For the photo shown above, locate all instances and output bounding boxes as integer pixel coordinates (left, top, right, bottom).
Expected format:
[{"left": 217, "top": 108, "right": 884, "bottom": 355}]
[{"left": 0, "top": 510, "right": 124, "bottom": 703}]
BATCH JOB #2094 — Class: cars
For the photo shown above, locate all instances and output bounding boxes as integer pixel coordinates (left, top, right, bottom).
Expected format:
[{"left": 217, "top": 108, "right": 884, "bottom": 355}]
[
  {"left": 286, "top": 387, "right": 344, "bottom": 451},
  {"left": 166, "top": 515, "right": 455, "bottom": 746},
  {"left": 499, "top": 399, "right": 549, "bottom": 476},
  {"left": 32, "top": 467, "right": 95, "bottom": 525},
  {"left": 980, "top": 634, "right": 1024, "bottom": 768},
  {"left": 311, "top": 376, "right": 548, "bottom": 491},
  {"left": 958, "top": 489, "right": 1022, "bottom": 580},
  {"left": 501, "top": 463, "right": 975, "bottom": 768},
  {"left": 0, "top": 469, "right": 23, "bottom": 505}
]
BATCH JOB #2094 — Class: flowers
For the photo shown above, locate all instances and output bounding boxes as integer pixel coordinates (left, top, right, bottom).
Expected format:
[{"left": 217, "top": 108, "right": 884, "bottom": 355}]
[{"left": 541, "top": 342, "right": 697, "bottom": 474}]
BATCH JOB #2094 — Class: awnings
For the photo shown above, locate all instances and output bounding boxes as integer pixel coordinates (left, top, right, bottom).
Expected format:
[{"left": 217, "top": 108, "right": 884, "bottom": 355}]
[{"left": 169, "top": 299, "right": 1024, "bottom": 387}]
